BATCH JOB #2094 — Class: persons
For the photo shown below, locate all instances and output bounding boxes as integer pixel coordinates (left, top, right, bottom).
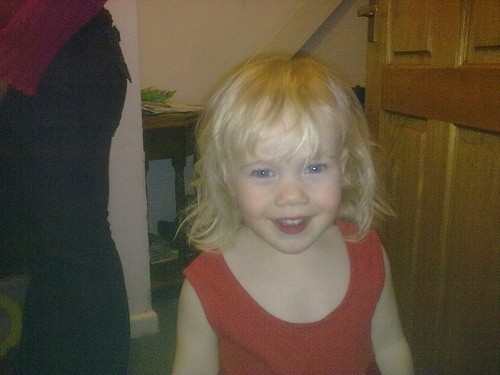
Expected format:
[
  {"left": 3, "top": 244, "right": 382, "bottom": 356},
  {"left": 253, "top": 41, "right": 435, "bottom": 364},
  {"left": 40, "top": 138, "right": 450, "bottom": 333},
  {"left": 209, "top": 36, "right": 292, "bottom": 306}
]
[
  {"left": 172, "top": 51, "right": 413, "bottom": 375},
  {"left": 0, "top": 0, "right": 132, "bottom": 375}
]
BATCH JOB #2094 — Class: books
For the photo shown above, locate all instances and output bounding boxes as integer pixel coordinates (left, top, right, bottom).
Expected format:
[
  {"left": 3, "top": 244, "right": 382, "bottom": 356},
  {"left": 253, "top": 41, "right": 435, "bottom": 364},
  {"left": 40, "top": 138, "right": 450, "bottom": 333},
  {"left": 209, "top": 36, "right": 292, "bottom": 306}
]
[{"left": 142, "top": 102, "right": 203, "bottom": 117}]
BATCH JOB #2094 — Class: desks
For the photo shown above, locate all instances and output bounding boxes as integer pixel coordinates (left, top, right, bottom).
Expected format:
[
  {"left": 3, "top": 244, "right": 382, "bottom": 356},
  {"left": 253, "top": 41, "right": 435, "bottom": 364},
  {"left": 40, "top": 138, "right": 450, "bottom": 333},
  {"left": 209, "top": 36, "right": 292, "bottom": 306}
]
[{"left": 143, "top": 103, "right": 205, "bottom": 238}]
